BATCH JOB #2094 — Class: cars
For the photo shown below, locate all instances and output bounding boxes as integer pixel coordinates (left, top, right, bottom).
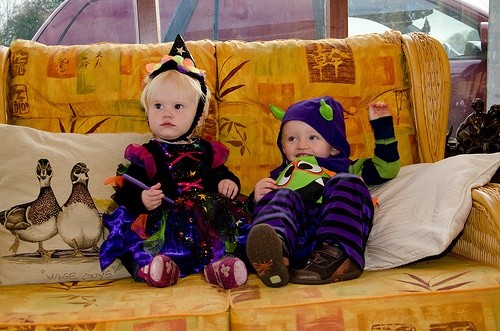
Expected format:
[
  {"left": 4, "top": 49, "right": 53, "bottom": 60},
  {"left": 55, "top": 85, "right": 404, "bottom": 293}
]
[{"left": 25, "top": 3, "right": 500, "bottom": 157}]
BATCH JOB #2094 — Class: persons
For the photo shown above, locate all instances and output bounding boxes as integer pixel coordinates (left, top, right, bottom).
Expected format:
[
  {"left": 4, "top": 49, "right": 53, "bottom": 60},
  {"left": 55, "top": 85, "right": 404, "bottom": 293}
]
[
  {"left": 454, "top": 98, "right": 500, "bottom": 154},
  {"left": 99, "top": 33, "right": 250, "bottom": 290},
  {"left": 243, "top": 95, "right": 400, "bottom": 289}
]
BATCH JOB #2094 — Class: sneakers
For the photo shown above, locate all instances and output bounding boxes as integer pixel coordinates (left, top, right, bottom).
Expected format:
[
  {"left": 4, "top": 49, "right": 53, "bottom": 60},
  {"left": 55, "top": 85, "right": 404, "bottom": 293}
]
[
  {"left": 288, "top": 244, "right": 363, "bottom": 285},
  {"left": 246, "top": 223, "right": 290, "bottom": 288}
]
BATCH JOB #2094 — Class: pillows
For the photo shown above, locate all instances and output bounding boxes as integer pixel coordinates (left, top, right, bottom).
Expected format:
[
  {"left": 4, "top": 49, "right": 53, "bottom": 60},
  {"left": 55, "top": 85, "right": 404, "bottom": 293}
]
[
  {"left": 363, "top": 152, "right": 500, "bottom": 271},
  {"left": 0, "top": 123, "right": 154, "bottom": 287}
]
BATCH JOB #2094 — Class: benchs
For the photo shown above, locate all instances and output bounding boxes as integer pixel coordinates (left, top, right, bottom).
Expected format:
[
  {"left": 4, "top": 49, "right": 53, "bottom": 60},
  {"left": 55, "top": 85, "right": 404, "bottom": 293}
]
[{"left": 0, "top": 32, "right": 500, "bottom": 331}]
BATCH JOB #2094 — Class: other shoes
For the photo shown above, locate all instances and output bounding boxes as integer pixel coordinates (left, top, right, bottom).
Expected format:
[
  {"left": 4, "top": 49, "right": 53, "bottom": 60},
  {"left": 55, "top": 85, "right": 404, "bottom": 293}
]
[
  {"left": 137, "top": 255, "right": 180, "bottom": 288},
  {"left": 204, "top": 256, "right": 248, "bottom": 290}
]
